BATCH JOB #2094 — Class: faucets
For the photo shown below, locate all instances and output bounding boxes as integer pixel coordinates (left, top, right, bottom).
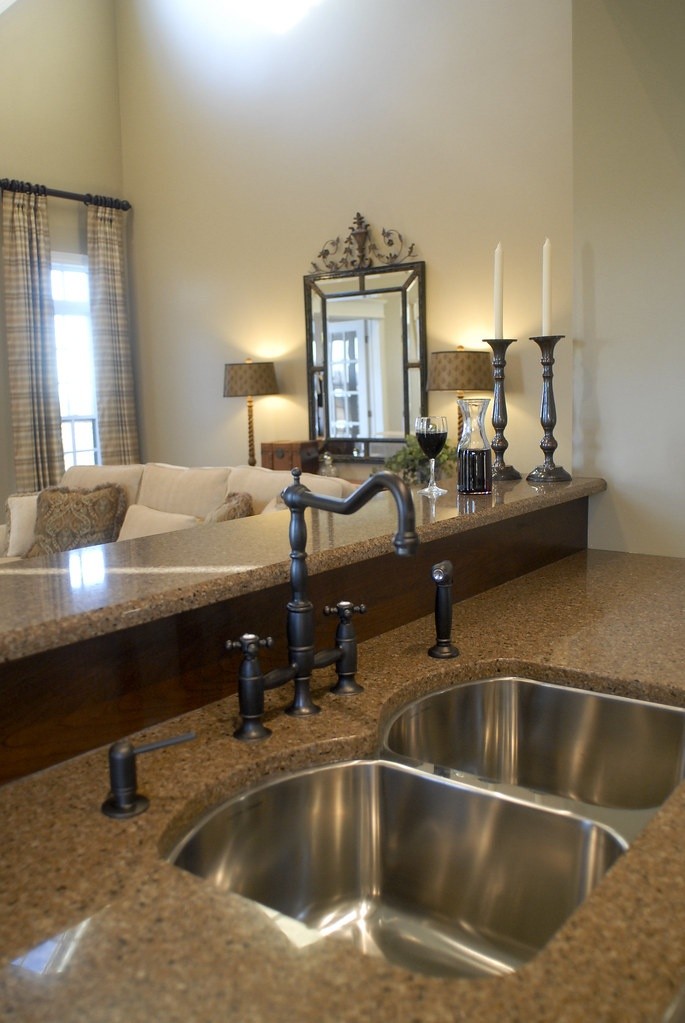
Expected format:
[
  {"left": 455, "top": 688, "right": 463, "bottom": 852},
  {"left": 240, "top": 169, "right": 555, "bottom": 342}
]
[{"left": 279, "top": 466, "right": 418, "bottom": 559}]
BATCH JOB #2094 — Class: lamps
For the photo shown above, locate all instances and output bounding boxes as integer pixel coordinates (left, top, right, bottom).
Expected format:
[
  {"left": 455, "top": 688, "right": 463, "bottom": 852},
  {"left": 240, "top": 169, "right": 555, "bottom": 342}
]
[
  {"left": 427, "top": 345, "right": 496, "bottom": 454},
  {"left": 223, "top": 358, "right": 280, "bottom": 468}
]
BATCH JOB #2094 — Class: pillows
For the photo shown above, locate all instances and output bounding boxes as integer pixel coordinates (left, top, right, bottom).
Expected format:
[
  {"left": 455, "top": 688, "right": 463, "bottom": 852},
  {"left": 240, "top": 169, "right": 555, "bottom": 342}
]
[
  {"left": 205, "top": 491, "right": 255, "bottom": 522},
  {"left": 5, "top": 492, "right": 38, "bottom": 556},
  {"left": 22, "top": 481, "right": 130, "bottom": 563},
  {"left": 116, "top": 503, "right": 200, "bottom": 543}
]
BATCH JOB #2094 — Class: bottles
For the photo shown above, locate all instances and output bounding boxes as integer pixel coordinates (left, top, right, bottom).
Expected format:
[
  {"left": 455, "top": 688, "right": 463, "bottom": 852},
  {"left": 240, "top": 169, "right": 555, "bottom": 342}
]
[{"left": 457, "top": 398, "right": 494, "bottom": 496}]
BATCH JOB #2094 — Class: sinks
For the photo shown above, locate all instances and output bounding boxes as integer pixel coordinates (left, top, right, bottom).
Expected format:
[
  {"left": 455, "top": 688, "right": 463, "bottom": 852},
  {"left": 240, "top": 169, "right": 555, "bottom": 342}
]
[
  {"left": 375, "top": 660, "right": 685, "bottom": 812},
  {"left": 158, "top": 734, "right": 628, "bottom": 975}
]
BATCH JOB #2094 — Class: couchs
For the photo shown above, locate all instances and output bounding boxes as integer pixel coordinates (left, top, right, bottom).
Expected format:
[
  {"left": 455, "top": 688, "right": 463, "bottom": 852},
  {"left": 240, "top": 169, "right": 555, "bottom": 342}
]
[{"left": 0, "top": 463, "right": 365, "bottom": 564}]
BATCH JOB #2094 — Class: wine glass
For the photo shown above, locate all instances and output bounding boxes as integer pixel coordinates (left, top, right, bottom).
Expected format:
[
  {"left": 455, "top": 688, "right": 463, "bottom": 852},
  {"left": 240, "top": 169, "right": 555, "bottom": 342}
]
[{"left": 415, "top": 416, "right": 449, "bottom": 498}]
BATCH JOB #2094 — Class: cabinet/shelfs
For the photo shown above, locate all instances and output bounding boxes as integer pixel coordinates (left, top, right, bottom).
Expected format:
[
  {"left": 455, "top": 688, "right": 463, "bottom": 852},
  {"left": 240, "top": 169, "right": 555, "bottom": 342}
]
[{"left": 261, "top": 442, "right": 320, "bottom": 474}]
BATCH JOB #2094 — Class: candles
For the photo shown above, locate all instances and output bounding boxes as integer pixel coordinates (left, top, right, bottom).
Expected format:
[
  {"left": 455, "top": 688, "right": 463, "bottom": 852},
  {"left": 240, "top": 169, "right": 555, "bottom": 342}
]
[
  {"left": 491, "top": 240, "right": 509, "bottom": 339},
  {"left": 540, "top": 236, "right": 555, "bottom": 337}
]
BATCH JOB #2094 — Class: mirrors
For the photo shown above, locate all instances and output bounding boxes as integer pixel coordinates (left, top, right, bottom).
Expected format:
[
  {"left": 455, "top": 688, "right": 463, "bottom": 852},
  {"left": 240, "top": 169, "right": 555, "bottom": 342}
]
[{"left": 303, "top": 211, "right": 428, "bottom": 465}]
